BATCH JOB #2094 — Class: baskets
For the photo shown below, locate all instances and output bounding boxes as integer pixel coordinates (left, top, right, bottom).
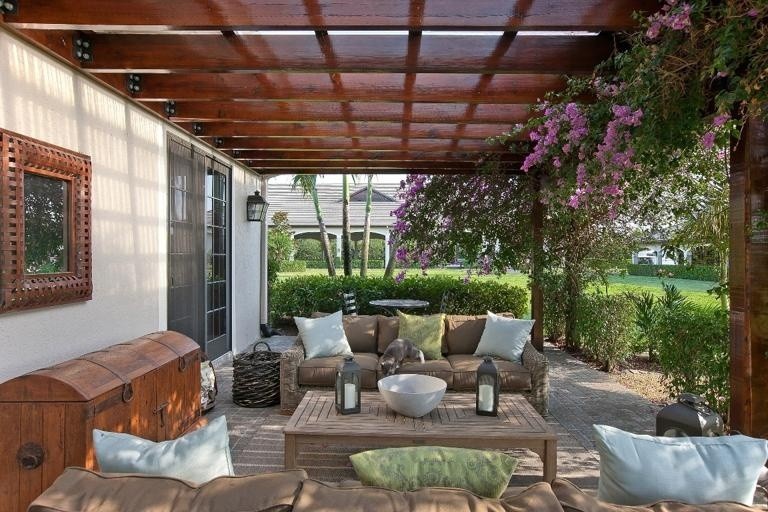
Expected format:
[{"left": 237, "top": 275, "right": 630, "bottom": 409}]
[{"left": 231, "top": 341, "right": 283, "bottom": 409}]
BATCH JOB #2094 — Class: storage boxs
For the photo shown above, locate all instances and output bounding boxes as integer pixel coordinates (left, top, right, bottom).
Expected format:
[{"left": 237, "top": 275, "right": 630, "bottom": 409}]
[{"left": 0, "top": 329, "right": 201, "bottom": 512}]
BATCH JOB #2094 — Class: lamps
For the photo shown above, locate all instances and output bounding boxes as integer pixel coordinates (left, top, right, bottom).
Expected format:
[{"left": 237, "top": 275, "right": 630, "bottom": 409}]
[
  {"left": 474, "top": 356, "right": 499, "bottom": 416},
  {"left": 657, "top": 393, "right": 725, "bottom": 437},
  {"left": 332, "top": 355, "right": 362, "bottom": 415},
  {"left": 246, "top": 189, "right": 270, "bottom": 221}
]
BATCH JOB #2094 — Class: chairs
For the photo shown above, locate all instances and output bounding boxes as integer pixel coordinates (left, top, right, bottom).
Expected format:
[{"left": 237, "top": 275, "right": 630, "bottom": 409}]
[
  {"left": 439, "top": 288, "right": 456, "bottom": 314},
  {"left": 341, "top": 292, "right": 361, "bottom": 315}
]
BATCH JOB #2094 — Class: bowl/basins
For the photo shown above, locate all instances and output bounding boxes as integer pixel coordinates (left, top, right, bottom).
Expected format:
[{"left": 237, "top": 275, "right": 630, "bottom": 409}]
[{"left": 378, "top": 374, "right": 448, "bottom": 418}]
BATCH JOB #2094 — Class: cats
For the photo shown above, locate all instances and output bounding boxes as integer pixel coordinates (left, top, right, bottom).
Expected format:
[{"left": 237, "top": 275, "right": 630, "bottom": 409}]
[{"left": 378, "top": 337, "right": 426, "bottom": 377}]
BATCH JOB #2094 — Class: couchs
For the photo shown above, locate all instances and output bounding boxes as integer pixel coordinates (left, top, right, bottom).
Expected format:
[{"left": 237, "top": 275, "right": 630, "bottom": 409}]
[
  {"left": 276, "top": 310, "right": 549, "bottom": 418},
  {"left": 23, "top": 462, "right": 768, "bottom": 512}
]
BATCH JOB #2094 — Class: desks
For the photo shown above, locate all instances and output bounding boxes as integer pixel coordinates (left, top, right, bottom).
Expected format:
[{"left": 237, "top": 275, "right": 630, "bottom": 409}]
[
  {"left": 279, "top": 387, "right": 560, "bottom": 486},
  {"left": 371, "top": 299, "right": 427, "bottom": 317}
]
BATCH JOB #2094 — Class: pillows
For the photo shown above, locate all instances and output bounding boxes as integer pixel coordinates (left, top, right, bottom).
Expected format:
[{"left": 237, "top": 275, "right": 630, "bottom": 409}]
[
  {"left": 396, "top": 309, "right": 448, "bottom": 360},
  {"left": 347, "top": 449, "right": 516, "bottom": 499},
  {"left": 291, "top": 309, "right": 353, "bottom": 359},
  {"left": 473, "top": 311, "right": 535, "bottom": 366},
  {"left": 90, "top": 412, "right": 235, "bottom": 484},
  {"left": 591, "top": 422, "right": 767, "bottom": 506}
]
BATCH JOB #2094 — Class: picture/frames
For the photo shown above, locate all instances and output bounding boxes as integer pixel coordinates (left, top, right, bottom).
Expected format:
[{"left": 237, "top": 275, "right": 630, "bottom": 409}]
[{"left": 0, "top": 127, "right": 95, "bottom": 317}]
